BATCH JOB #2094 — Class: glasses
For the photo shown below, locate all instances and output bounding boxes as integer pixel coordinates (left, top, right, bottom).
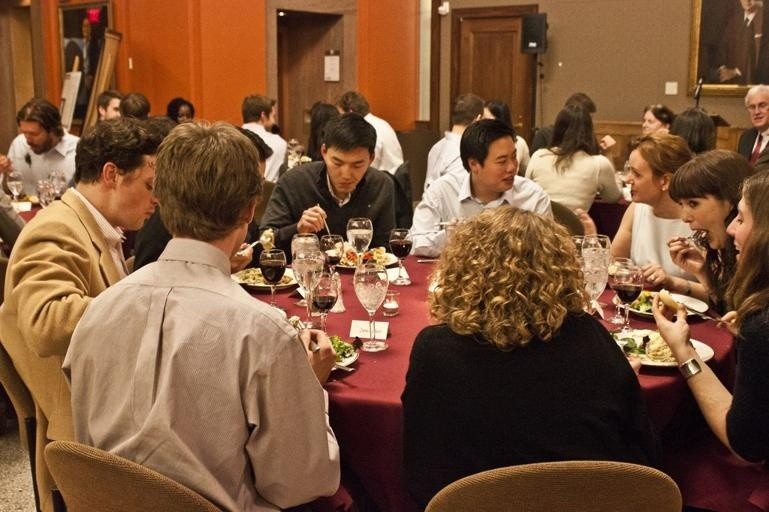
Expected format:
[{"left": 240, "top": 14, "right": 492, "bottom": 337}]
[{"left": 745, "top": 105, "right": 769, "bottom": 112}]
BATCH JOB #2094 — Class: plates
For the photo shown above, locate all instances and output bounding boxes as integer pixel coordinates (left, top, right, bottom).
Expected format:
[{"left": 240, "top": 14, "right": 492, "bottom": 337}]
[
  {"left": 611, "top": 290, "right": 715, "bottom": 367},
  {"left": 230, "top": 266, "right": 303, "bottom": 294},
  {"left": 331, "top": 338, "right": 360, "bottom": 373}
]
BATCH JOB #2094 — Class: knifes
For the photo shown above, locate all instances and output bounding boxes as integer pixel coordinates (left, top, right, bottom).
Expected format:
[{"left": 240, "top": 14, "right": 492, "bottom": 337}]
[{"left": 678, "top": 302, "right": 725, "bottom": 322}]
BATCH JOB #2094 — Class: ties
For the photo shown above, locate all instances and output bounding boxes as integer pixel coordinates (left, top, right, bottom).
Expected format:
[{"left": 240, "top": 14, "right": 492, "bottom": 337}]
[{"left": 748, "top": 132, "right": 764, "bottom": 166}]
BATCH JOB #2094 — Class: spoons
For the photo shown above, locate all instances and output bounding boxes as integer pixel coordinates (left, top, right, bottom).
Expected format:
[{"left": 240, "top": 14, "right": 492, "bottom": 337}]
[{"left": 662, "top": 230, "right": 710, "bottom": 244}]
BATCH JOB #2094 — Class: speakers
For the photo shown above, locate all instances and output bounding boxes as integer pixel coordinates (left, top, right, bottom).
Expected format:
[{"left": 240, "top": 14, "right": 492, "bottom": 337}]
[{"left": 521, "top": 12, "right": 547, "bottom": 54}]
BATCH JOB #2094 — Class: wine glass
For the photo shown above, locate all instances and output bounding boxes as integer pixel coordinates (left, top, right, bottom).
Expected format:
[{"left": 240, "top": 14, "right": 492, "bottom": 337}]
[
  {"left": 567, "top": 233, "right": 645, "bottom": 340},
  {"left": 6, "top": 170, "right": 67, "bottom": 209},
  {"left": 258, "top": 212, "right": 415, "bottom": 353}
]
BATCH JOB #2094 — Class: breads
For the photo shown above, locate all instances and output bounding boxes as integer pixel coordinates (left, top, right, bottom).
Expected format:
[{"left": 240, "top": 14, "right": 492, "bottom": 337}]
[{"left": 660, "top": 288, "right": 688, "bottom": 314}]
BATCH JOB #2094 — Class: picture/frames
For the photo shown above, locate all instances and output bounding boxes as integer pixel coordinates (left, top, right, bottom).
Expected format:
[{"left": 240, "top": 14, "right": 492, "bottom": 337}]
[
  {"left": 685, "top": 0, "right": 769, "bottom": 98},
  {"left": 57, "top": 0, "right": 118, "bottom": 125}
]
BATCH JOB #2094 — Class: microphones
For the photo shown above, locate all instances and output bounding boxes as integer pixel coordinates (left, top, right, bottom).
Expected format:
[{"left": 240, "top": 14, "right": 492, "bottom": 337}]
[{"left": 693, "top": 77, "right": 703, "bottom": 99}]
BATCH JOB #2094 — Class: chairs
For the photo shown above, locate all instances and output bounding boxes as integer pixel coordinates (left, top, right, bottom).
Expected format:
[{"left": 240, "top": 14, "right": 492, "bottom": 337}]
[
  {"left": 0, "top": 258, "right": 44, "bottom": 512},
  {"left": 421, "top": 459, "right": 684, "bottom": 512},
  {"left": 42, "top": 439, "right": 223, "bottom": 511}
]
